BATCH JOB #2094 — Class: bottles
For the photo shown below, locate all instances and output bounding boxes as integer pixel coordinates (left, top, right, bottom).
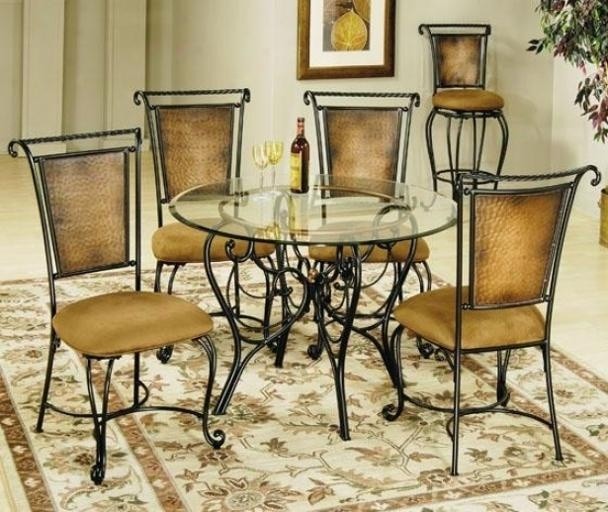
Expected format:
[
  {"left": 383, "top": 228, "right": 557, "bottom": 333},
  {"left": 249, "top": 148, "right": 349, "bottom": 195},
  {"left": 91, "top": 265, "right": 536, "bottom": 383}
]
[{"left": 291, "top": 115, "right": 310, "bottom": 194}]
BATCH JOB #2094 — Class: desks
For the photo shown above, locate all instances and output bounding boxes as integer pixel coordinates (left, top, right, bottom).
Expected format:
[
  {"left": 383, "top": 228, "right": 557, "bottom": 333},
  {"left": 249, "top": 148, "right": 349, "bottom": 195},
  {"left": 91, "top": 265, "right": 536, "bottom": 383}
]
[{"left": 168, "top": 174, "right": 457, "bottom": 441}]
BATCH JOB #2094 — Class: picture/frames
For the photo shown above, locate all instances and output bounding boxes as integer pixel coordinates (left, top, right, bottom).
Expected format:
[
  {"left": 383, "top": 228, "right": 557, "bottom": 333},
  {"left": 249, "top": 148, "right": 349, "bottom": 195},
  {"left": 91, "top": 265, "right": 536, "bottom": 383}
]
[{"left": 297, "top": 0, "right": 395, "bottom": 80}]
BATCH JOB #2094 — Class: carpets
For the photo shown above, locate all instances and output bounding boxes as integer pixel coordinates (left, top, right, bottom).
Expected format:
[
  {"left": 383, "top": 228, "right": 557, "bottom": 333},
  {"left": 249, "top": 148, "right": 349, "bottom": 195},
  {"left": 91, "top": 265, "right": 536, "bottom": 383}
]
[{"left": 0, "top": 253, "right": 608, "bottom": 512}]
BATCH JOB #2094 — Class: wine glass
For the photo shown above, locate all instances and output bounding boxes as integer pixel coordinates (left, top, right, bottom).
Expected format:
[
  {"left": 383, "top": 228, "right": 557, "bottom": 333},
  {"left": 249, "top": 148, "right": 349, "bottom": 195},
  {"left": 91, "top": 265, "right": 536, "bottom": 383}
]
[
  {"left": 252, "top": 144, "right": 270, "bottom": 199},
  {"left": 265, "top": 139, "right": 284, "bottom": 196}
]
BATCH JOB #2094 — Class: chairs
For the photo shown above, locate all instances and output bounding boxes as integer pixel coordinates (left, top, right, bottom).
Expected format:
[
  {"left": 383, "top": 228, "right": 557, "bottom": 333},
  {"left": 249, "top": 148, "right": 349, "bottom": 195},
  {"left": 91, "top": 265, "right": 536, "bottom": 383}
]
[
  {"left": 133, "top": 88, "right": 279, "bottom": 363},
  {"left": 9, "top": 128, "right": 226, "bottom": 486},
  {"left": 304, "top": 91, "right": 434, "bottom": 361},
  {"left": 382, "top": 163, "right": 601, "bottom": 476},
  {"left": 418, "top": 24, "right": 509, "bottom": 202}
]
